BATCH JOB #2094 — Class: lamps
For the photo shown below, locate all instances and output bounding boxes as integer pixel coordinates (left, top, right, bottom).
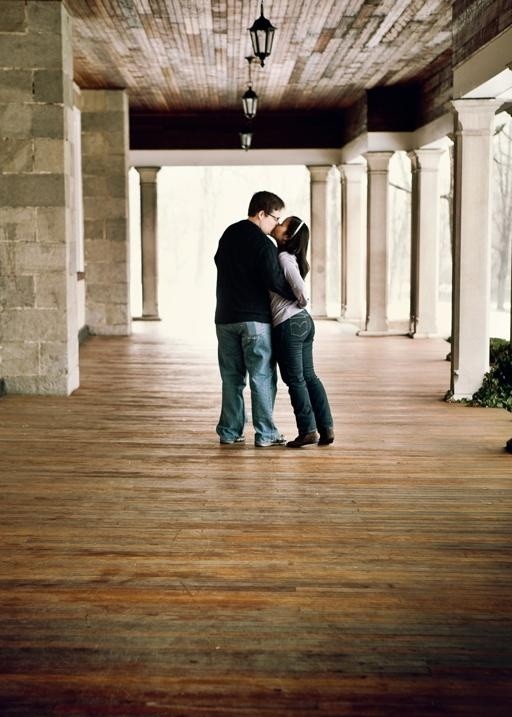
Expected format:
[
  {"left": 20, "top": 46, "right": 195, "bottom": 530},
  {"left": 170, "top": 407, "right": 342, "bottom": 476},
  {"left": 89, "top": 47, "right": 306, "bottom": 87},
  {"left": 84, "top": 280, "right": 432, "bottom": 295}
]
[
  {"left": 241, "top": 57, "right": 258, "bottom": 119},
  {"left": 239, "top": 125, "right": 253, "bottom": 151},
  {"left": 246, "top": 0, "right": 278, "bottom": 68}
]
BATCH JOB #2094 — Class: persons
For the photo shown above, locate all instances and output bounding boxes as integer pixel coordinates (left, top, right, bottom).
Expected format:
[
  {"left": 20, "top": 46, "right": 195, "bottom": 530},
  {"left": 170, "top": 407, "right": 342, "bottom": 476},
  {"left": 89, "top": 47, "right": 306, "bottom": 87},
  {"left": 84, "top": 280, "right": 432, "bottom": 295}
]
[
  {"left": 266, "top": 214, "right": 335, "bottom": 448},
  {"left": 212, "top": 189, "right": 299, "bottom": 448}
]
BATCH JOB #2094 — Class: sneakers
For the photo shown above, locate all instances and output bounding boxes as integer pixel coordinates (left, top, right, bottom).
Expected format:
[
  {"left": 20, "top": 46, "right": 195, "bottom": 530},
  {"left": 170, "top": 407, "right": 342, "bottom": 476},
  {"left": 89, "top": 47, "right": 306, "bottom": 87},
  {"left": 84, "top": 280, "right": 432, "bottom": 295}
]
[
  {"left": 287, "top": 431, "right": 319, "bottom": 446},
  {"left": 318, "top": 428, "right": 335, "bottom": 445},
  {"left": 255, "top": 434, "right": 287, "bottom": 447},
  {"left": 220, "top": 434, "right": 245, "bottom": 444}
]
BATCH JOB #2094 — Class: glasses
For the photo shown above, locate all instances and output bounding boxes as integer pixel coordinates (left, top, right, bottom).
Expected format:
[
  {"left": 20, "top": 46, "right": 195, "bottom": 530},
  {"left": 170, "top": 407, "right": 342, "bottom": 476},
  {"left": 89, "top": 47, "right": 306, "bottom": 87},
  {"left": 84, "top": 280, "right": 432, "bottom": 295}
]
[{"left": 267, "top": 212, "right": 280, "bottom": 221}]
